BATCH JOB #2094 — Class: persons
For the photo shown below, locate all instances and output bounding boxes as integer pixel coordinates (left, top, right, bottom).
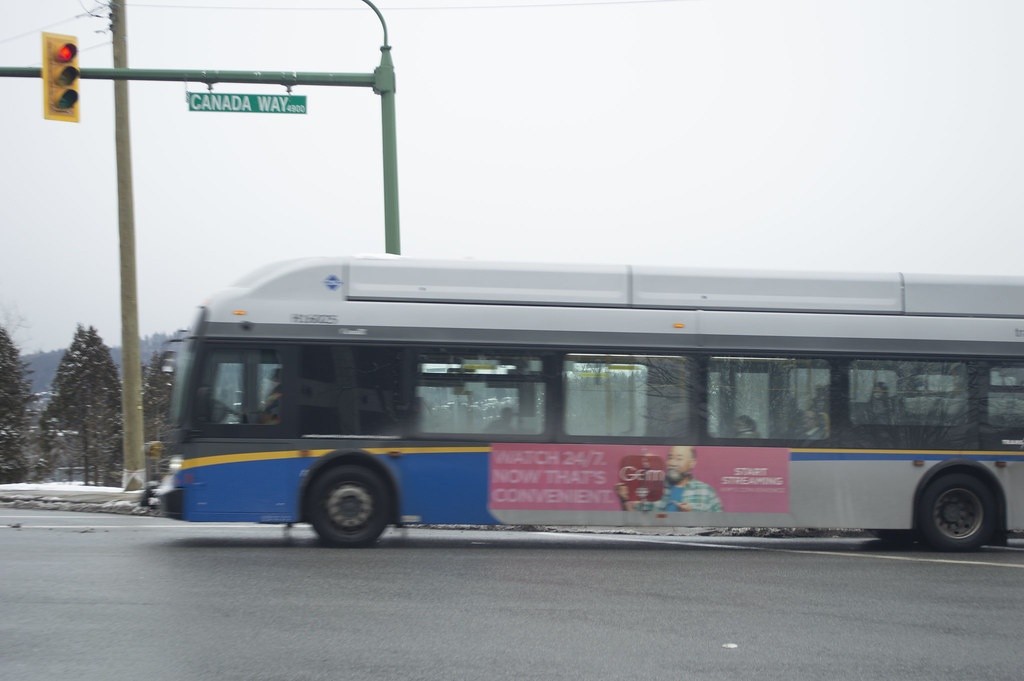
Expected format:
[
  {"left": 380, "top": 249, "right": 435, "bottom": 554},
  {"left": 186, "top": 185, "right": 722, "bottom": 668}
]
[
  {"left": 777, "top": 382, "right": 891, "bottom": 440},
  {"left": 614, "top": 446, "right": 724, "bottom": 512},
  {"left": 731, "top": 415, "right": 762, "bottom": 439},
  {"left": 243, "top": 368, "right": 281, "bottom": 425}
]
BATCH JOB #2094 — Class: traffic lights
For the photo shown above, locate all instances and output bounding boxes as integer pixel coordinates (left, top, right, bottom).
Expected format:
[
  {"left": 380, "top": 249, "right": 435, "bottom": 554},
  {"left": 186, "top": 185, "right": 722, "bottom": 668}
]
[{"left": 40, "top": 32, "right": 87, "bottom": 124}]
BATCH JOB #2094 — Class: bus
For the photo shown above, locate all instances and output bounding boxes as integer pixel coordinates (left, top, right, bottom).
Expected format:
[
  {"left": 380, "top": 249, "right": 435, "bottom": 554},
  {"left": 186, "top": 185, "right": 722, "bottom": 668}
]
[{"left": 135, "top": 250, "right": 1024, "bottom": 546}]
